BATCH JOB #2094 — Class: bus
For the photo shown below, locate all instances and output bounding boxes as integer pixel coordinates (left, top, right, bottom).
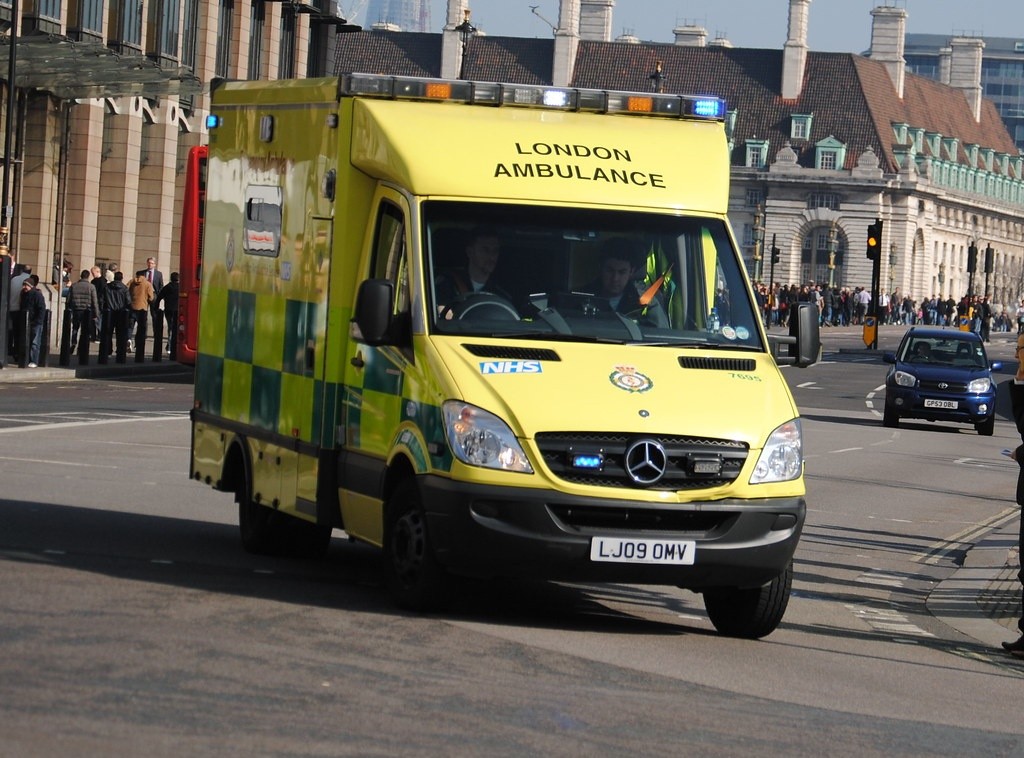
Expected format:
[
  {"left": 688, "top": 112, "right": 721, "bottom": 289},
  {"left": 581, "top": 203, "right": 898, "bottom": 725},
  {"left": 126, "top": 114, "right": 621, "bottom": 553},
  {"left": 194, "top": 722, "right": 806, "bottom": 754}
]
[{"left": 176, "top": 146, "right": 208, "bottom": 363}]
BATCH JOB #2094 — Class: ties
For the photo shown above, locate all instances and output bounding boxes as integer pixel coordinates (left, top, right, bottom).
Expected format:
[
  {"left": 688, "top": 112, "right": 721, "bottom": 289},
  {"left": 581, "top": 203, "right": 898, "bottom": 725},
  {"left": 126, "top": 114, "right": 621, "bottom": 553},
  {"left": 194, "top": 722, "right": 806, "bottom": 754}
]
[
  {"left": 881, "top": 295, "right": 883, "bottom": 305},
  {"left": 148, "top": 271, "right": 152, "bottom": 283}
]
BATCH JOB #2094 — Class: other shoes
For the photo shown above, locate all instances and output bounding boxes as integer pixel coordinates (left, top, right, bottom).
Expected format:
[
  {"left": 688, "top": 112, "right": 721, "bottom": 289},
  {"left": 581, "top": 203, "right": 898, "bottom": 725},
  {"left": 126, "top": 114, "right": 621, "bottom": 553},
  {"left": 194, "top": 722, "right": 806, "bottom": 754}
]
[
  {"left": 884, "top": 322, "right": 886, "bottom": 325},
  {"left": 167, "top": 351, "right": 170, "bottom": 354},
  {"left": 897, "top": 321, "right": 900, "bottom": 324},
  {"left": 1002, "top": 635, "right": 1024, "bottom": 654},
  {"left": 127, "top": 339, "right": 131, "bottom": 353},
  {"left": 70, "top": 345, "right": 75, "bottom": 354},
  {"left": 893, "top": 322, "right": 896, "bottom": 325},
  {"left": 28, "top": 363, "right": 37, "bottom": 367}
]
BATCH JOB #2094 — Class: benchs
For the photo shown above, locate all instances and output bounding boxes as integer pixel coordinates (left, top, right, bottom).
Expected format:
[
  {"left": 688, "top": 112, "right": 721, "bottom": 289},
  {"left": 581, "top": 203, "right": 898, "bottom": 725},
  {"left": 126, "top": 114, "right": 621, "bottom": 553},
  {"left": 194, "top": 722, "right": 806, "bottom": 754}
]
[{"left": 931, "top": 349, "right": 961, "bottom": 360}]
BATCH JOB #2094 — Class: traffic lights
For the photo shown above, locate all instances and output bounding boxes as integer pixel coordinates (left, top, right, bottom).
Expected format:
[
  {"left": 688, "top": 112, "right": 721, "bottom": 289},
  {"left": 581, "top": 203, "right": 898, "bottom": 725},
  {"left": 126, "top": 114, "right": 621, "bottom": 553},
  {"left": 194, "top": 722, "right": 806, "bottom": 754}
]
[
  {"left": 770, "top": 246, "right": 781, "bottom": 263},
  {"left": 866, "top": 225, "right": 878, "bottom": 260}
]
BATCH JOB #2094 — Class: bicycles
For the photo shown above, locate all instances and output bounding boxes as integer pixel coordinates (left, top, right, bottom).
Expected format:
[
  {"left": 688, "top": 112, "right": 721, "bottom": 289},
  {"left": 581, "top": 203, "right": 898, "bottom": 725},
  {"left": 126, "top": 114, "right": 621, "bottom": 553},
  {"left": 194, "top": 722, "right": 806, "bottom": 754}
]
[{"left": 1016, "top": 316, "right": 1024, "bottom": 335}]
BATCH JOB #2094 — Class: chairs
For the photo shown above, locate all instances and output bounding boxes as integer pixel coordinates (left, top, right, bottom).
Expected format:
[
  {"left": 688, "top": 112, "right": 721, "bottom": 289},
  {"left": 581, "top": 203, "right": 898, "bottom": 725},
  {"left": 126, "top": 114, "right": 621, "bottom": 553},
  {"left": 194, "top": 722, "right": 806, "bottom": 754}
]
[
  {"left": 953, "top": 344, "right": 978, "bottom": 364},
  {"left": 432, "top": 228, "right": 468, "bottom": 301},
  {"left": 909, "top": 342, "right": 935, "bottom": 362}
]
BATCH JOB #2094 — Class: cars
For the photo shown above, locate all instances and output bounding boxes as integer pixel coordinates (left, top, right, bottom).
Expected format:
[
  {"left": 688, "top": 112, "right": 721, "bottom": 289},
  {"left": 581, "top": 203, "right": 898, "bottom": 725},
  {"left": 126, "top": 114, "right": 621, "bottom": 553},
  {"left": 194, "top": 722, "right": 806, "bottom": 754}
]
[{"left": 882, "top": 325, "right": 1004, "bottom": 437}]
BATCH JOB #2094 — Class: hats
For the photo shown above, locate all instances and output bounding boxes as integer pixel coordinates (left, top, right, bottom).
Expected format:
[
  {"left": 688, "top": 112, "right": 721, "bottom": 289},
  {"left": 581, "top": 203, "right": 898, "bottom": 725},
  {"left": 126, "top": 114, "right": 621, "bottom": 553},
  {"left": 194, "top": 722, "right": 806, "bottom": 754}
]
[{"left": 23, "top": 278, "right": 34, "bottom": 288}]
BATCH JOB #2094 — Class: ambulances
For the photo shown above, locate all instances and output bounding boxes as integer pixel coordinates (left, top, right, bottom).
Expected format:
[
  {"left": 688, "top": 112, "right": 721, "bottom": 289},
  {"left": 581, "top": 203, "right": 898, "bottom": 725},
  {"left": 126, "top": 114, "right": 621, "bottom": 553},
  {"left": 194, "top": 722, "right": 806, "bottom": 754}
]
[{"left": 188, "top": 74, "right": 818, "bottom": 640}]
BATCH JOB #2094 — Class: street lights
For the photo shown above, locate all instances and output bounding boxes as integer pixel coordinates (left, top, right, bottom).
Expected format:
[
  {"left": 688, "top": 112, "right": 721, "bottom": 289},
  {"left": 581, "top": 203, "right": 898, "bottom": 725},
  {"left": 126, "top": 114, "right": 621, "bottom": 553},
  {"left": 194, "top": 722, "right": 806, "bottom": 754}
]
[
  {"left": 454, "top": 10, "right": 478, "bottom": 79},
  {"left": 752, "top": 205, "right": 767, "bottom": 282},
  {"left": 826, "top": 224, "right": 840, "bottom": 289},
  {"left": 889, "top": 243, "right": 898, "bottom": 293},
  {"left": 938, "top": 262, "right": 947, "bottom": 300}
]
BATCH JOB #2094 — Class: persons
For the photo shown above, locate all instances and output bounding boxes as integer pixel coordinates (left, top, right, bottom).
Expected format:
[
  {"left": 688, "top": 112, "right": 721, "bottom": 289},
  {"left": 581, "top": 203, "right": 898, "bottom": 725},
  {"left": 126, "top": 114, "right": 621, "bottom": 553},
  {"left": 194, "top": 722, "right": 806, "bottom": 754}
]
[
  {"left": 429, "top": 227, "right": 518, "bottom": 320},
  {"left": 0, "top": 243, "right": 180, "bottom": 368},
  {"left": 1002, "top": 334, "right": 1024, "bottom": 653},
  {"left": 749, "top": 279, "right": 1024, "bottom": 363},
  {"left": 565, "top": 241, "right": 672, "bottom": 328}
]
